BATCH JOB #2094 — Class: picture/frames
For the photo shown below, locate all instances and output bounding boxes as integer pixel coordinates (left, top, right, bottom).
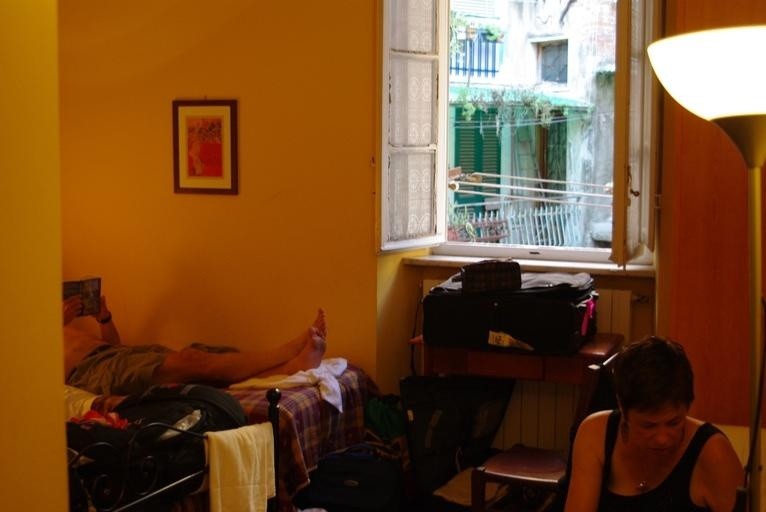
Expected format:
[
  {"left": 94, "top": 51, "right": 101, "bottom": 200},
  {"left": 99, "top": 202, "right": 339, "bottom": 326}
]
[{"left": 173, "top": 98, "right": 237, "bottom": 195}]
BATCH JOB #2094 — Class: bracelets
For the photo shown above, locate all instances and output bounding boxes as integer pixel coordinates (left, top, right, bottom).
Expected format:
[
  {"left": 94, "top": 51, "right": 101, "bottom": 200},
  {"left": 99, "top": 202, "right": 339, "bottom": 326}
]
[{"left": 97, "top": 312, "right": 112, "bottom": 327}]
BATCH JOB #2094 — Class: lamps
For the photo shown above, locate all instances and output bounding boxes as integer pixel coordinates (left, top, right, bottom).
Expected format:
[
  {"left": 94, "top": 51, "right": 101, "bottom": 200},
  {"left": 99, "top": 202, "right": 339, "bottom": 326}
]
[{"left": 647, "top": 24, "right": 766, "bottom": 512}]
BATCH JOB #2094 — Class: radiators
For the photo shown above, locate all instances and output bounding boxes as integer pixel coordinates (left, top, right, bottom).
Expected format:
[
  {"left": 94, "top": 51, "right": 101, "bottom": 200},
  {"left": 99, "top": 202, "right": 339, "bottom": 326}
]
[{"left": 418, "top": 279, "right": 647, "bottom": 451}]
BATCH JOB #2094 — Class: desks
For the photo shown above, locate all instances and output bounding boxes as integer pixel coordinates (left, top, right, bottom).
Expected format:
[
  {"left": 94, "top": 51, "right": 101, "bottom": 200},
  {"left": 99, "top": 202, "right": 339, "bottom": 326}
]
[{"left": 407, "top": 334, "right": 625, "bottom": 384}]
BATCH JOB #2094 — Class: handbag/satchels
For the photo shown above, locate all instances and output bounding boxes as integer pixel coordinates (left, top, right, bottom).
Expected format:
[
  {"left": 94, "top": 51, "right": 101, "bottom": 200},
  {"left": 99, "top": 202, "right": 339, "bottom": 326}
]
[
  {"left": 312, "top": 443, "right": 414, "bottom": 512},
  {"left": 110, "top": 383, "right": 247, "bottom": 473}
]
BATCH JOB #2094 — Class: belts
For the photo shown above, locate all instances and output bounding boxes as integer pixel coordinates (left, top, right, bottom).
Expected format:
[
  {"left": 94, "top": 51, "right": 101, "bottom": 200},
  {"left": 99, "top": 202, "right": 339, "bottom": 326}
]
[{"left": 65, "top": 344, "right": 110, "bottom": 383}]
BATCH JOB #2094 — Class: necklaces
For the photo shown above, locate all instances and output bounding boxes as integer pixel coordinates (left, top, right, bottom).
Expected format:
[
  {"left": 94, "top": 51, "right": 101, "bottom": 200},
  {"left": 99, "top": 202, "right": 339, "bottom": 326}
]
[{"left": 627, "top": 444, "right": 665, "bottom": 491}]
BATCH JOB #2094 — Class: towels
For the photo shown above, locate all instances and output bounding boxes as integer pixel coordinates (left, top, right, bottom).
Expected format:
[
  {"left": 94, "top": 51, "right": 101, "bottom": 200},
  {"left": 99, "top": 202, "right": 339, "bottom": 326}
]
[
  {"left": 228, "top": 357, "right": 347, "bottom": 413},
  {"left": 203, "top": 422, "right": 276, "bottom": 512}
]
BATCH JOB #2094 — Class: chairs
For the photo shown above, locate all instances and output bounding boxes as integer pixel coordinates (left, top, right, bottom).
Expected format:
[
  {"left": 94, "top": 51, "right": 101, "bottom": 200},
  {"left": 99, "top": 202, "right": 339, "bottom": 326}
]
[{"left": 471, "top": 340, "right": 641, "bottom": 512}]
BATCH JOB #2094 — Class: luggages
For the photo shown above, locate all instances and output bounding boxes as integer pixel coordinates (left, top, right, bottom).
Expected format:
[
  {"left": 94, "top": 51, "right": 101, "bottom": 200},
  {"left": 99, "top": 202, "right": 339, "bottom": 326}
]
[{"left": 421, "top": 272, "right": 595, "bottom": 354}]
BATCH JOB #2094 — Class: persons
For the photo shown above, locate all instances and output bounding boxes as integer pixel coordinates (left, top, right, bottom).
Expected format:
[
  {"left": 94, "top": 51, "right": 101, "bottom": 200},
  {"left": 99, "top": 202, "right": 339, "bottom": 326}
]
[
  {"left": 63, "top": 293, "right": 327, "bottom": 397},
  {"left": 563, "top": 336, "right": 750, "bottom": 512}
]
